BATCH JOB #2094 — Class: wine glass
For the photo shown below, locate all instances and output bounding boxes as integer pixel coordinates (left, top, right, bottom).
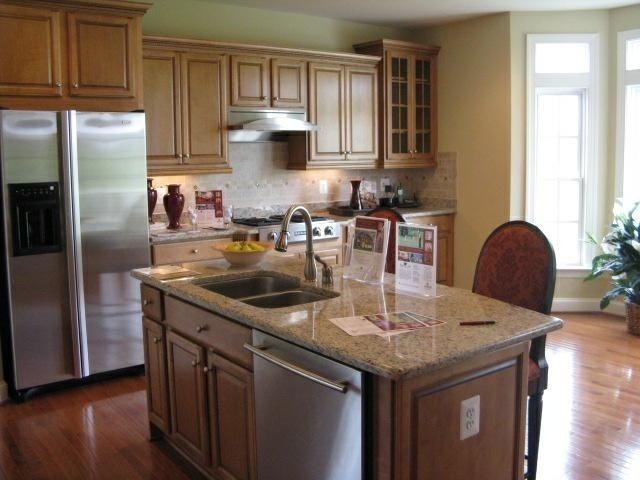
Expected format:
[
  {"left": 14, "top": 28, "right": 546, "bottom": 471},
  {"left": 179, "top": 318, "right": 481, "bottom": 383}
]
[
  {"left": 222, "top": 204, "right": 233, "bottom": 233},
  {"left": 186, "top": 206, "right": 200, "bottom": 233}
]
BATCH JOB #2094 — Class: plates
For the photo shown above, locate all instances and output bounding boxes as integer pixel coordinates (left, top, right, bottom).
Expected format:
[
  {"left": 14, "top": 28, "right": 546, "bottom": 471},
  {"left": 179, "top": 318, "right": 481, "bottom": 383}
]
[{"left": 213, "top": 226, "right": 231, "bottom": 230}]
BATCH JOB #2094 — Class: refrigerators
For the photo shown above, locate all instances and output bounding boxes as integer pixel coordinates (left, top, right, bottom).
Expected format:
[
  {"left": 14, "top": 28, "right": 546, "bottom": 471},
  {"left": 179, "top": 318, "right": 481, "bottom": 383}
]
[{"left": 0, "top": 109, "right": 152, "bottom": 389}]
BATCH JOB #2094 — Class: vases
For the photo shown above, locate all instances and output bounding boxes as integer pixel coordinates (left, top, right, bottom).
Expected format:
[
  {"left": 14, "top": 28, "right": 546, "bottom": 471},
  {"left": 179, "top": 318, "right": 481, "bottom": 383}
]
[
  {"left": 163, "top": 184, "right": 185, "bottom": 230},
  {"left": 350, "top": 180, "right": 361, "bottom": 210},
  {"left": 147, "top": 178, "right": 157, "bottom": 223}
]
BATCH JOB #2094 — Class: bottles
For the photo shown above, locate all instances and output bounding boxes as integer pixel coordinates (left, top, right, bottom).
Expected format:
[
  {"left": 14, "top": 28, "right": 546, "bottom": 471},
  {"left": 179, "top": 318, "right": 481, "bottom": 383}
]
[
  {"left": 394, "top": 186, "right": 405, "bottom": 204},
  {"left": 385, "top": 185, "right": 395, "bottom": 205}
]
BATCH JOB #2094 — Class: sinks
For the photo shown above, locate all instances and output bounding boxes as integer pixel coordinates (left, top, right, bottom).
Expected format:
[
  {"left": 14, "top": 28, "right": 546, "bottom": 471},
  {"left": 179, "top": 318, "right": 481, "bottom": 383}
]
[
  {"left": 194, "top": 268, "right": 299, "bottom": 299},
  {"left": 241, "top": 284, "right": 333, "bottom": 310}
]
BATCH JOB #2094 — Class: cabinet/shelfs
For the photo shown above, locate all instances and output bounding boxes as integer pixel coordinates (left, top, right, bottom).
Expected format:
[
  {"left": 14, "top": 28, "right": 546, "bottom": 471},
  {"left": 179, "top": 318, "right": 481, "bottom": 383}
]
[
  {"left": 228, "top": 42, "right": 307, "bottom": 118},
  {"left": 283, "top": 238, "right": 343, "bottom": 266},
  {"left": 353, "top": 38, "right": 440, "bottom": 169},
  {"left": 153, "top": 238, "right": 233, "bottom": 264},
  {"left": 164, "top": 293, "right": 257, "bottom": 480},
  {"left": 286, "top": 47, "right": 381, "bottom": 171},
  {"left": 142, "top": 35, "right": 232, "bottom": 173},
  {"left": 140, "top": 283, "right": 169, "bottom": 443},
  {"left": 408, "top": 214, "right": 454, "bottom": 287},
  {"left": 0, "top": 0, "right": 152, "bottom": 112}
]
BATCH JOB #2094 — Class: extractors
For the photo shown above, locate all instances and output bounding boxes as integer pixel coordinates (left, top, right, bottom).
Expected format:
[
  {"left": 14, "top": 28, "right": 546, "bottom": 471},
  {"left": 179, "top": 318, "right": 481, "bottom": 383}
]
[{"left": 229, "top": 112, "right": 319, "bottom": 144}]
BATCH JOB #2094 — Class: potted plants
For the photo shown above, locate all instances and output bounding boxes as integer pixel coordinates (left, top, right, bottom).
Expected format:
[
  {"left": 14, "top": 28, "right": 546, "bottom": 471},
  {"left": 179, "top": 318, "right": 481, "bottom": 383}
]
[{"left": 581, "top": 201, "right": 640, "bottom": 336}]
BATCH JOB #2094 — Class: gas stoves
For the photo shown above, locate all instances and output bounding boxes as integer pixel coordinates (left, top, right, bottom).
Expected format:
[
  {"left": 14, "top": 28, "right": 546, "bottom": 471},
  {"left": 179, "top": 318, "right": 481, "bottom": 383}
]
[{"left": 233, "top": 213, "right": 337, "bottom": 231}]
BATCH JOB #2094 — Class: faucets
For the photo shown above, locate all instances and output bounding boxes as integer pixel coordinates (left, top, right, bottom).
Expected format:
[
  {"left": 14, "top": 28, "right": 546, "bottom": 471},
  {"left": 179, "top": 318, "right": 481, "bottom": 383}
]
[{"left": 276, "top": 206, "right": 318, "bottom": 277}]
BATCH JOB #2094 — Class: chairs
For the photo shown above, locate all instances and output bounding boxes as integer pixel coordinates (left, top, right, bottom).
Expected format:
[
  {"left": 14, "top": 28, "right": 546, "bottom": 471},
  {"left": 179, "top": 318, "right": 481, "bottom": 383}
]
[
  {"left": 366, "top": 208, "right": 405, "bottom": 274},
  {"left": 471, "top": 220, "right": 557, "bottom": 479}
]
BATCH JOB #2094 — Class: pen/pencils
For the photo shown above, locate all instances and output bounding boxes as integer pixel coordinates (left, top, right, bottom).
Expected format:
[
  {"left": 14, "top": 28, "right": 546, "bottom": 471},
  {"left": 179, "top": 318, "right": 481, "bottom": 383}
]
[{"left": 460, "top": 320, "right": 495, "bottom": 325}]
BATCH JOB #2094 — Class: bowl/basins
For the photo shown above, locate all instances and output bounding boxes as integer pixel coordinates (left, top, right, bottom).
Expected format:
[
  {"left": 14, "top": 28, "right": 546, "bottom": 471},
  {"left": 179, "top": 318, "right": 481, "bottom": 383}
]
[{"left": 212, "top": 242, "right": 274, "bottom": 266}]
